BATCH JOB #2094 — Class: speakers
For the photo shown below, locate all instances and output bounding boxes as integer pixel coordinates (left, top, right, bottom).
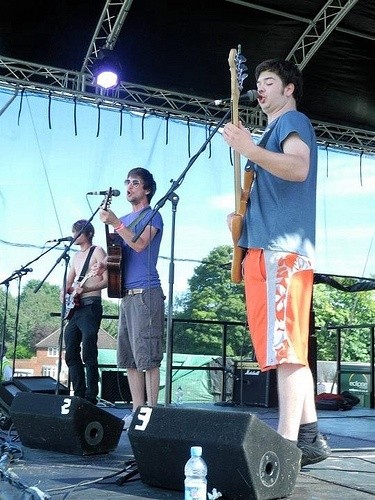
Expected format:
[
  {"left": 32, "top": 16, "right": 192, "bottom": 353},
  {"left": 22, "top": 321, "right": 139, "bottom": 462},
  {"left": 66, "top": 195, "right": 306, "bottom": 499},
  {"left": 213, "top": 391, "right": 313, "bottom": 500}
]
[
  {"left": 101, "top": 370, "right": 148, "bottom": 402},
  {"left": 232, "top": 361, "right": 279, "bottom": 407},
  {"left": 0, "top": 376, "right": 125, "bottom": 456},
  {"left": 127, "top": 405, "right": 303, "bottom": 500}
]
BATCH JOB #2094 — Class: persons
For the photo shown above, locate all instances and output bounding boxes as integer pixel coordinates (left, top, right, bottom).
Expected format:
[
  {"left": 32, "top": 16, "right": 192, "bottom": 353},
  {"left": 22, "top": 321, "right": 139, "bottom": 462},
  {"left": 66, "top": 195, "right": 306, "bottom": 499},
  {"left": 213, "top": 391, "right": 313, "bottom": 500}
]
[
  {"left": 0, "top": 342, "right": 12, "bottom": 382},
  {"left": 221, "top": 58, "right": 330, "bottom": 468},
  {"left": 99, "top": 167, "right": 165, "bottom": 430},
  {"left": 60, "top": 220, "right": 108, "bottom": 406}
]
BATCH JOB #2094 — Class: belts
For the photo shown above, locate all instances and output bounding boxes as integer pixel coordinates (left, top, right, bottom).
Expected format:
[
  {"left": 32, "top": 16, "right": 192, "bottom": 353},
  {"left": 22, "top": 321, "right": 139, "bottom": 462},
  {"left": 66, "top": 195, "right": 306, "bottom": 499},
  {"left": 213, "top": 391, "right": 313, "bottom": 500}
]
[
  {"left": 80, "top": 300, "right": 101, "bottom": 306},
  {"left": 126, "top": 287, "right": 145, "bottom": 294}
]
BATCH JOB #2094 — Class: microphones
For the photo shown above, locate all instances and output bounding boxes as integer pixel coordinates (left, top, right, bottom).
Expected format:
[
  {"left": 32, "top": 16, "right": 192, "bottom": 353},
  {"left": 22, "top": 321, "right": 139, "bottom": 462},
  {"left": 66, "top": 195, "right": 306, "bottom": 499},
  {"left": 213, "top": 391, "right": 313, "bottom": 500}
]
[
  {"left": 15, "top": 268, "right": 33, "bottom": 272},
  {"left": 211, "top": 89, "right": 259, "bottom": 106},
  {"left": 87, "top": 190, "right": 121, "bottom": 197},
  {"left": 48, "top": 236, "right": 73, "bottom": 242},
  {"left": 237, "top": 379, "right": 250, "bottom": 384}
]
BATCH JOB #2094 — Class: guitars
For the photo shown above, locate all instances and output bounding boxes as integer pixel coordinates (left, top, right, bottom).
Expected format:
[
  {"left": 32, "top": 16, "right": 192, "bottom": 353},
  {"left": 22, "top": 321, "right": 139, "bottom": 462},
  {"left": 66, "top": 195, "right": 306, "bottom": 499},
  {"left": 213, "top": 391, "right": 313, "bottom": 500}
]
[
  {"left": 63, "top": 270, "right": 98, "bottom": 321},
  {"left": 226, "top": 43, "right": 257, "bottom": 285},
  {"left": 102, "top": 186, "right": 125, "bottom": 299}
]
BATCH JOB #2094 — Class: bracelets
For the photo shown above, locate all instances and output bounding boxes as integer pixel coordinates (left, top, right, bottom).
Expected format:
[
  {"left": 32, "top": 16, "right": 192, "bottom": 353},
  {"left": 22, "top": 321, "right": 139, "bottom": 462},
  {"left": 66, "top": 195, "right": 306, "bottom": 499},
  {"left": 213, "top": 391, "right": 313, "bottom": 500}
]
[{"left": 113, "top": 221, "right": 125, "bottom": 233}]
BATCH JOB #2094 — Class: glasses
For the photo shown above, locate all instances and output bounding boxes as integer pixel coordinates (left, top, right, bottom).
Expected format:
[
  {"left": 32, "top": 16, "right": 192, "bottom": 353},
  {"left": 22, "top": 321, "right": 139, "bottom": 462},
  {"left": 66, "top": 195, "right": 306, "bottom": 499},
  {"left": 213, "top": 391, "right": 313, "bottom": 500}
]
[{"left": 123, "top": 179, "right": 144, "bottom": 186}]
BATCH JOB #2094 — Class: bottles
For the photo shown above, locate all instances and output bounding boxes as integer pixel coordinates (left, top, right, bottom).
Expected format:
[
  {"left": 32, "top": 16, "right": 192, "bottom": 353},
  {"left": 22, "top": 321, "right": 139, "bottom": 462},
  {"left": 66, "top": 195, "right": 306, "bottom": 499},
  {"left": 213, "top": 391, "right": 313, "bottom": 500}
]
[
  {"left": 0, "top": 448, "right": 13, "bottom": 471},
  {"left": 184, "top": 447, "right": 207, "bottom": 500},
  {"left": 177, "top": 386, "right": 183, "bottom": 404}
]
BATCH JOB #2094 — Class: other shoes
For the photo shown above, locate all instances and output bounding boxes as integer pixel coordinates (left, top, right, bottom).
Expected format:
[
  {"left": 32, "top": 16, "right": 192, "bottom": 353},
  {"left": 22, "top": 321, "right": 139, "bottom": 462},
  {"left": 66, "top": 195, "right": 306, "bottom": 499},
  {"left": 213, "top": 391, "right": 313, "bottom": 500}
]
[
  {"left": 122, "top": 413, "right": 133, "bottom": 430},
  {"left": 297, "top": 433, "right": 332, "bottom": 467}
]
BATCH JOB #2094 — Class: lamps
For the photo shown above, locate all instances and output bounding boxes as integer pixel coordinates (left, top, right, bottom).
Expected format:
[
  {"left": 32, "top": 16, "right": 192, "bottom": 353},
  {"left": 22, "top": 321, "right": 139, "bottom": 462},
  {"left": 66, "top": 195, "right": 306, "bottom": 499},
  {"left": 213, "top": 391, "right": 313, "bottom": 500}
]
[{"left": 92, "top": 46, "right": 121, "bottom": 88}]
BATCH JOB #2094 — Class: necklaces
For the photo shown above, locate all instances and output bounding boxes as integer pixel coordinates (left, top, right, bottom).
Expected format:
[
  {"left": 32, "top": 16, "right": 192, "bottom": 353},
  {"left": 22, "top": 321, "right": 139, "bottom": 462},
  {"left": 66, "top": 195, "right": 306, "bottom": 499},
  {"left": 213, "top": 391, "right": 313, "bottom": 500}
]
[{"left": 79, "top": 244, "right": 91, "bottom": 252}]
